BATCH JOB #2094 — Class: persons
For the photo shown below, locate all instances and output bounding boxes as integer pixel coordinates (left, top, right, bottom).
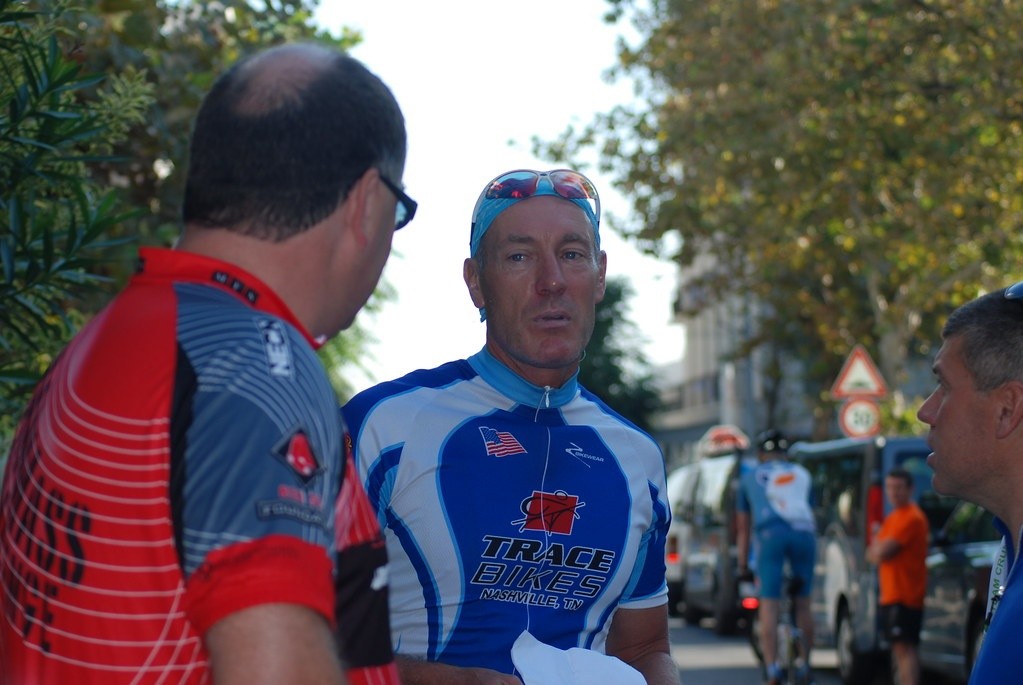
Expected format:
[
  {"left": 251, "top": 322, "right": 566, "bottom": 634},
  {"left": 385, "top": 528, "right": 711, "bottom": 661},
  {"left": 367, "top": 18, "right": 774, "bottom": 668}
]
[
  {"left": 861, "top": 468, "right": 928, "bottom": 684},
  {"left": 339, "top": 167, "right": 682, "bottom": 685},
  {"left": 915, "top": 282, "right": 1023, "bottom": 685},
  {"left": 0, "top": 43, "right": 406, "bottom": 685},
  {"left": 732, "top": 428, "right": 818, "bottom": 685}
]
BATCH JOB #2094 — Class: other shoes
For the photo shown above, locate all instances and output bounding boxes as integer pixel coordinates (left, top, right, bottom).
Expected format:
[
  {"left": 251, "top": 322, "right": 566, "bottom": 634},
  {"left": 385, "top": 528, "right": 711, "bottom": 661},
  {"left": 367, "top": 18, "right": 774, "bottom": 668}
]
[
  {"left": 792, "top": 657, "right": 810, "bottom": 685},
  {"left": 767, "top": 661, "right": 782, "bottom": 685}
]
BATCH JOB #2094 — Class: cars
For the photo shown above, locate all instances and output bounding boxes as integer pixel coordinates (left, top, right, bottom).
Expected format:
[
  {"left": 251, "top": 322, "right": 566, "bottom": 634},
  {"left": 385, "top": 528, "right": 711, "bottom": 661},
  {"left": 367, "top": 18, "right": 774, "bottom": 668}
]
[
  {"left": 834, "top": 502, "right": 1007, "bottom": 685},
  {"left": 664, "top": 438, "right": 952, "bottom": 643}
]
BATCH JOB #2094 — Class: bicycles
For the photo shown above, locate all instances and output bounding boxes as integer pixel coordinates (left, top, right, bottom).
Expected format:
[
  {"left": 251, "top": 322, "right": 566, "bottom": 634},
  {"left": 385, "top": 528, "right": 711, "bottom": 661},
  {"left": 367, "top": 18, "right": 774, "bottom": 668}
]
[{"left": 732, "top": 566, "right": 798, "bottom": 685}]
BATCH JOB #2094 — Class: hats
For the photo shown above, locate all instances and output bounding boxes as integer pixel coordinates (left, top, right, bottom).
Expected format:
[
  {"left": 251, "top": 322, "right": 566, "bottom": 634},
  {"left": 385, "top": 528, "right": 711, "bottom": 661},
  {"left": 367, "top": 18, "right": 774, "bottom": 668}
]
[
  {"left": 469, "top": 169, "right": 603, "bottom": 323},
  {"left": 757, "top": 429, "right": 789, "bottom": 452}
]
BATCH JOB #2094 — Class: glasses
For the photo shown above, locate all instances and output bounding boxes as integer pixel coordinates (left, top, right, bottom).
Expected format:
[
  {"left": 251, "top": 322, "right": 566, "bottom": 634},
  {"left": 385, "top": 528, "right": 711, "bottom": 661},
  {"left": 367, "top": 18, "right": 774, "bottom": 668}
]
[
  {"left": 348, "top": 161, "right": 417, "bottom": 230},
  {"left": 1005, "top": 280, "right": 1023, "bottom": 300},
  {"left": 470, "top": 169, "right": 601, "bottom": 257}
]
